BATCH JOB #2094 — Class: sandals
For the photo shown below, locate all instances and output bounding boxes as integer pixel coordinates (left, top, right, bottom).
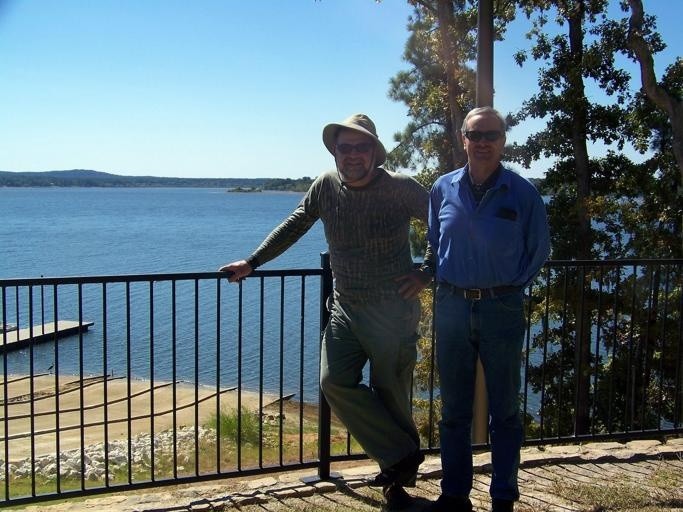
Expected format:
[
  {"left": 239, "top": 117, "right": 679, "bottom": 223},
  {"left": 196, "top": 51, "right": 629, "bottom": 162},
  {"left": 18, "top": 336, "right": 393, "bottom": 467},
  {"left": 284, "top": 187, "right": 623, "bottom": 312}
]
[
  {"left": 367, "top": 449, "right": 425, "bottom": 486},
  {"left": 383, "top": 485, "right": 416, "bottom": 511}
]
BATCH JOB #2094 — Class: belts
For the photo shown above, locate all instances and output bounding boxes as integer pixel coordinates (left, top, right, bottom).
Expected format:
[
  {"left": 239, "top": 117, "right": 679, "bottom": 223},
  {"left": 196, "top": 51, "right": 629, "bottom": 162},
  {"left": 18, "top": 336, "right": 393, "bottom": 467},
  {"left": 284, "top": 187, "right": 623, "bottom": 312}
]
[{"left": 439, "top": 279, "right": 523, "bottom": 300}]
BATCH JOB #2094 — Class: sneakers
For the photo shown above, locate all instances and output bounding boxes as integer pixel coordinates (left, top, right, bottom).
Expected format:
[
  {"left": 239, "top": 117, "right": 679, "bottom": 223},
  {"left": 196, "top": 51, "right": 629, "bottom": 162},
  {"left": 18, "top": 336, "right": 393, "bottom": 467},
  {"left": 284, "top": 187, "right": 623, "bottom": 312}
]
[
  {"left": 420, "top": 494, "right": 472, "bottom": 512},
  {"left": 493, "top": 498, "right": 514, "bottom": 511}
]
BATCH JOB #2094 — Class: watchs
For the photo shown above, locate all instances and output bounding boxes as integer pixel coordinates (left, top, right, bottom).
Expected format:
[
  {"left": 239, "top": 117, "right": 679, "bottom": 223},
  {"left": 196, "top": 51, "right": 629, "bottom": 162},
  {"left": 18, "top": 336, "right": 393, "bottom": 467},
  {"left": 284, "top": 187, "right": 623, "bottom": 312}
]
[{"left": 420, "top": 263, "right": 432, "bottom": 275}]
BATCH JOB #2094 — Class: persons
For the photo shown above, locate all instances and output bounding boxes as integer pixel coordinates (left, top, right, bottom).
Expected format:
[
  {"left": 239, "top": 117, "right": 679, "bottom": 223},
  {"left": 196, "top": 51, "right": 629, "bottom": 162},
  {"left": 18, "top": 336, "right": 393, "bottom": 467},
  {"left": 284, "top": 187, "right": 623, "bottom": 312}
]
[
  {"left": 427, "top": 108, "right": 551, "bottom": 509},
  {"left": 218, "top": 113, "right": 439, "bottom": 508}
]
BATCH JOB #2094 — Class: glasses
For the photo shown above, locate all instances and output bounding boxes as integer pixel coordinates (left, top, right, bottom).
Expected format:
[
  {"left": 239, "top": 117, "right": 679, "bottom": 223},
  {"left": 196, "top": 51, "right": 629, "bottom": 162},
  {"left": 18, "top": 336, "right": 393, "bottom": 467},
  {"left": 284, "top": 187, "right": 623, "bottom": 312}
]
[
  {"left": 464, "top": 131, "right": 505, "bottom": 141},
  {"left": 334, "top": 143, "right": 371, "bottom": 152}
]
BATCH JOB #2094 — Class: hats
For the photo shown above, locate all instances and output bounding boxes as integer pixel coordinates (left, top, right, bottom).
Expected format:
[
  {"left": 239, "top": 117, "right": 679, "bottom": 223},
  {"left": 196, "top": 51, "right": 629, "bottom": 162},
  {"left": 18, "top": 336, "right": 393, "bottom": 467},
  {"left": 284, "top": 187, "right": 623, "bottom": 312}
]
[{"left": 323, "top": 114, "right": 386, "bottom": 167}]
